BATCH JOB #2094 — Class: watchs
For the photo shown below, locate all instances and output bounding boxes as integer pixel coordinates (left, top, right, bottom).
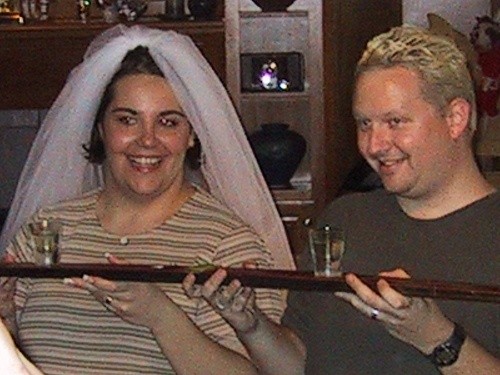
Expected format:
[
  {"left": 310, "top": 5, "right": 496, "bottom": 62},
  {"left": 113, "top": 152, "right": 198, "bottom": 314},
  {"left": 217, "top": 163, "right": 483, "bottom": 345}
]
[{"left": 424, "top": 321, "right": 468, "bottom": 367}]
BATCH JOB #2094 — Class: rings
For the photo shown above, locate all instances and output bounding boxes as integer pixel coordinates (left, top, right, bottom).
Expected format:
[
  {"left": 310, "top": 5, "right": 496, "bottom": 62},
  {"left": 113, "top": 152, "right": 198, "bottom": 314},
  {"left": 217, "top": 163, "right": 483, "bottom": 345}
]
[
  {"left": 104, "top": 295, "right": 113, "bottom": 304},
  {"left": 370, "top": 306, "right": 379, "bottom": 321}
]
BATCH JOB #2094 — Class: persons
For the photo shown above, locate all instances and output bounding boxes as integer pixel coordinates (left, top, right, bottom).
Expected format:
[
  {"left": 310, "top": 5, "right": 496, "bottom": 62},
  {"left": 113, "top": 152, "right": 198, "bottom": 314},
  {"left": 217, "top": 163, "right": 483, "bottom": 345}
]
[
  {"left": 0, "top": 26, "right": 290, "bottom": 373},
  {"left": 181, "top": 24, "right": 500, "bottom": 375}
]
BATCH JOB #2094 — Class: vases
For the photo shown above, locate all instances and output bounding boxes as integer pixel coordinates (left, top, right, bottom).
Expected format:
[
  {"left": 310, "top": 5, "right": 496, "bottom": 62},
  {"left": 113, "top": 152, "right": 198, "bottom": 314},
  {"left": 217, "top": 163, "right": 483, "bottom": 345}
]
[{"left": 247, "top": 123, "right": 307, "bottom": 189}]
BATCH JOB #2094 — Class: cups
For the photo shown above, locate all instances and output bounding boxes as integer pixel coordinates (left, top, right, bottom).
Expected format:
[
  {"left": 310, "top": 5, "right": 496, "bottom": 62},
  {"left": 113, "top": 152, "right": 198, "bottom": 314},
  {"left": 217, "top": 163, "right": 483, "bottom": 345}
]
[
  {"left": 27, "top": 221, "right": 62, "bottom": 267},
  {"left": 308, "top": 224, "right": 346, "bottom": 278}
]
